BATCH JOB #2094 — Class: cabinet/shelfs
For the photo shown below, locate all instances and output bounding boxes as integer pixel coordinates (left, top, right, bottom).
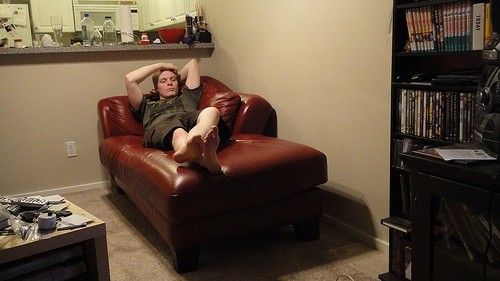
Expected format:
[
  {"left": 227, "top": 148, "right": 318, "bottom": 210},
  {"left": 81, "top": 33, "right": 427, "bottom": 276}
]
[
  {"left": 136, "top": 0, "right": 198, "bottom": 32},
  {"left": 29, "top": 0, "right": 76, "bottom": 33},
  {"left": 379, "top": 0, "right": 500, "bottom": 281},
  {"left": 73, "top": 0, "right": 139, "bottom": 31}
]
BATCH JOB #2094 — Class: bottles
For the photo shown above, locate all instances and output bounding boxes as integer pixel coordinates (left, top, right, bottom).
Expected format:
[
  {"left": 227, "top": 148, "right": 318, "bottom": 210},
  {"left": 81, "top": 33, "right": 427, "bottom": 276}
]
[
  {"left": 185, "top": 16, "right": 212, "bottom": 44},
  {"left": 140, "top": 32, "right": 150, "bottom": 44},
  {"left": 81, "top": 13, "right": 95, "bottom": 47},
  {"left": 103, "top": 16, "right": 117, "bottom": 46},
  {"left": 14, "top": 38, "right": 22, "bottom": 49}
]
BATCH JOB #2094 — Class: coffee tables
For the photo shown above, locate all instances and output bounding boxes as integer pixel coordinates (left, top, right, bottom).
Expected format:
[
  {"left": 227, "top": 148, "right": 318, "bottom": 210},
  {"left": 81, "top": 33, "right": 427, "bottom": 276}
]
[{"left": 0, "top": 195, "right": 110, "bottom": 281}]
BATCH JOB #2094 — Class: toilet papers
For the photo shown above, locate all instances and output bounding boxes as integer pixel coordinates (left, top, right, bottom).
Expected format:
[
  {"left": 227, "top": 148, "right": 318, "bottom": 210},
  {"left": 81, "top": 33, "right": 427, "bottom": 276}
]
[{"left": 118, "top": 5, "right": 135, "bottom": 43}]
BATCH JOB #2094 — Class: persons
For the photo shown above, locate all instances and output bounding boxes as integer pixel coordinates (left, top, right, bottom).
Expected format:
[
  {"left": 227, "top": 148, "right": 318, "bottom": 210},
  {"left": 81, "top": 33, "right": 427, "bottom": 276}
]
[{"left": 124, "top": 58, "right": 221, "bottom": 176}]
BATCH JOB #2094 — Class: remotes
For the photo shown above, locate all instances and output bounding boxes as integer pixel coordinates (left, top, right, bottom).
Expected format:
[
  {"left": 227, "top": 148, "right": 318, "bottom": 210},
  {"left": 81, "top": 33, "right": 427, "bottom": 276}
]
[{"left": 0, "top": 196, "right": 49, "bottom": 208}]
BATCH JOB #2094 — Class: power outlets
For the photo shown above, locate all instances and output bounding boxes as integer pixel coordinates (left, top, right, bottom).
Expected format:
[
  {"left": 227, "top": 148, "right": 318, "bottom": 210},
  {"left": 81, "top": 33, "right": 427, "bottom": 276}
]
[{"left": 66, "top": 142, "right": 77, "bottom": 157}]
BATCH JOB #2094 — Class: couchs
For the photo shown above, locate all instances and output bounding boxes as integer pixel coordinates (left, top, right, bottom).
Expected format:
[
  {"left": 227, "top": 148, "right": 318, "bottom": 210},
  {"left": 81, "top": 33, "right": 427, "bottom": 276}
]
[{"left": 98, "top": 76, "right": 328, "bottom": 274}]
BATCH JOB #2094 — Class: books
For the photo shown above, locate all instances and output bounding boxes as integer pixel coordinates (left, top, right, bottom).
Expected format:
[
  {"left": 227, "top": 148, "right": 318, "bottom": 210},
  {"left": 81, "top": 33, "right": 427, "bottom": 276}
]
[
  {"left": 397, "top": 88, "right": 475, "bottom": 143},
  {"left": 404, "top": 2, "right": 492, "bottom": 53},
  {"left": 381, "top": 172, "right": 500, "bottom": 281}
]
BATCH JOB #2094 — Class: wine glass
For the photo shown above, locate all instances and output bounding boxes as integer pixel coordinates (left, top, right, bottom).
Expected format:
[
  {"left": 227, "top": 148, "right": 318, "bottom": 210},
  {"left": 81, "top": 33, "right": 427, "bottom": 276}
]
[{"left": 50, "top": 16, "right": 63, "bottom": 47}]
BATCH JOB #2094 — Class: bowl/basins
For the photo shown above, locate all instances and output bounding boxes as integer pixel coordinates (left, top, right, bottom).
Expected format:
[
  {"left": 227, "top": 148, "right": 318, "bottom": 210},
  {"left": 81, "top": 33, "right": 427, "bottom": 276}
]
[{"left": 158, "top": 27, "right": 185, "bottom": 44}]
